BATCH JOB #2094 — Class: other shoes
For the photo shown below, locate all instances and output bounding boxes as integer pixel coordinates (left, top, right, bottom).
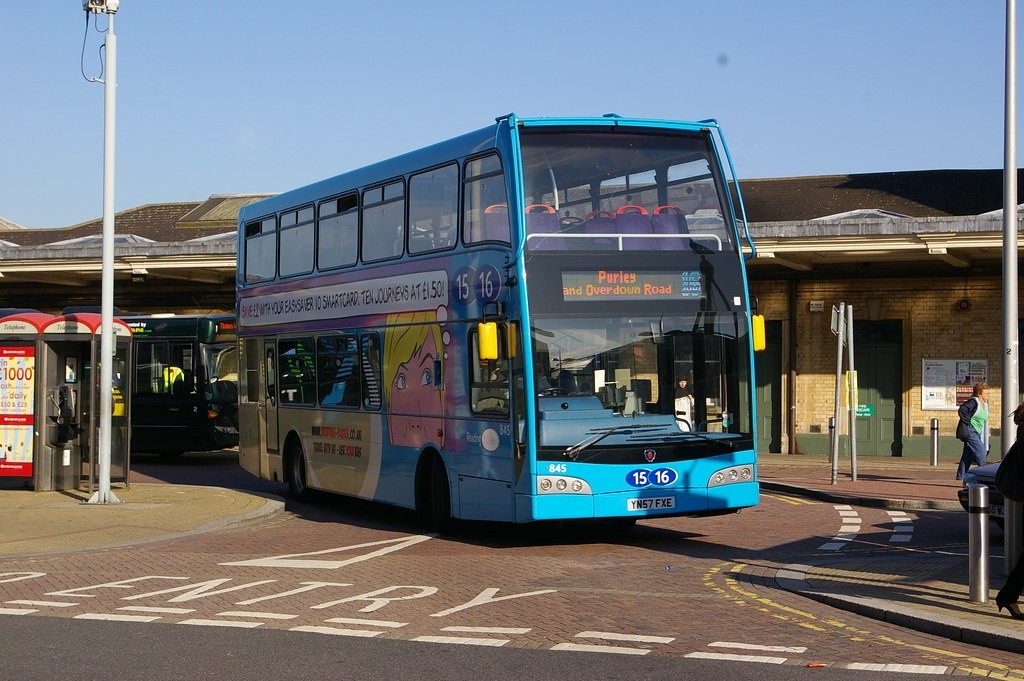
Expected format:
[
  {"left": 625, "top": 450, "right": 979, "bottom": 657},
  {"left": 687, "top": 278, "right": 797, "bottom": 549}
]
[{"left": 956, "top": 477, "right": 961, "bottom": 480}]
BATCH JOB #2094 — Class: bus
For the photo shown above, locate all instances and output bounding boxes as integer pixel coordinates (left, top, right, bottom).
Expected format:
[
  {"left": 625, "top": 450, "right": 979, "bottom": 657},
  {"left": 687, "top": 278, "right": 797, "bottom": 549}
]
[
  {"left": 0, "top": 307, "right": 241, "bottom": 456},
  {"left": 232, "top": 107, "right": 769, "bottom": 531}
]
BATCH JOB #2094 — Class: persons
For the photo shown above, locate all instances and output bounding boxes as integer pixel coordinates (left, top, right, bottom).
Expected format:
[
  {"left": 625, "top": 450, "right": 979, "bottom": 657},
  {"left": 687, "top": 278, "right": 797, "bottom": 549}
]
[
  {"left": 955, "top": 381, "right": 990, "bottom": 480},
  {"left": 995, "top": 400, "right": 1024, "bottom": 620},
  {"left": 675, "top": 375, "right": 694, "bottom": 432}
]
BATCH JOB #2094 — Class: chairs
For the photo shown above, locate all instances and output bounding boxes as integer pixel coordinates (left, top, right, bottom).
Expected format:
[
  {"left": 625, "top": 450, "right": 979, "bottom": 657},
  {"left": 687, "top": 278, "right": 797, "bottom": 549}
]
[
  {"left": 651, "top": 205, "right": 693, "bottom": 250},
  {"left": 398, "top": 197, "right": 615, "bottom": 256},
  {"left": 613, "top": 206, "right": 659, "bottom": 250}
]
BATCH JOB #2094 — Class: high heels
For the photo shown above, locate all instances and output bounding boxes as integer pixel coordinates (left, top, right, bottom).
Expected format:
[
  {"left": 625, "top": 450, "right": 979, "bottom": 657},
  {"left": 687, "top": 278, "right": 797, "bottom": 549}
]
[{"left": 996, "top": 597, "right": 1024, "bottom": 620}]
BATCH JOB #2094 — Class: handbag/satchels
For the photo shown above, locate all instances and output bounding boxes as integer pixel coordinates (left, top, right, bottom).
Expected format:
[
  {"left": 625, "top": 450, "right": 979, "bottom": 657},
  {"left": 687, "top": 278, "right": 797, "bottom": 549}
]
[{"left": 996, "top": 440, "right": 1024, "bottom": 501}]
